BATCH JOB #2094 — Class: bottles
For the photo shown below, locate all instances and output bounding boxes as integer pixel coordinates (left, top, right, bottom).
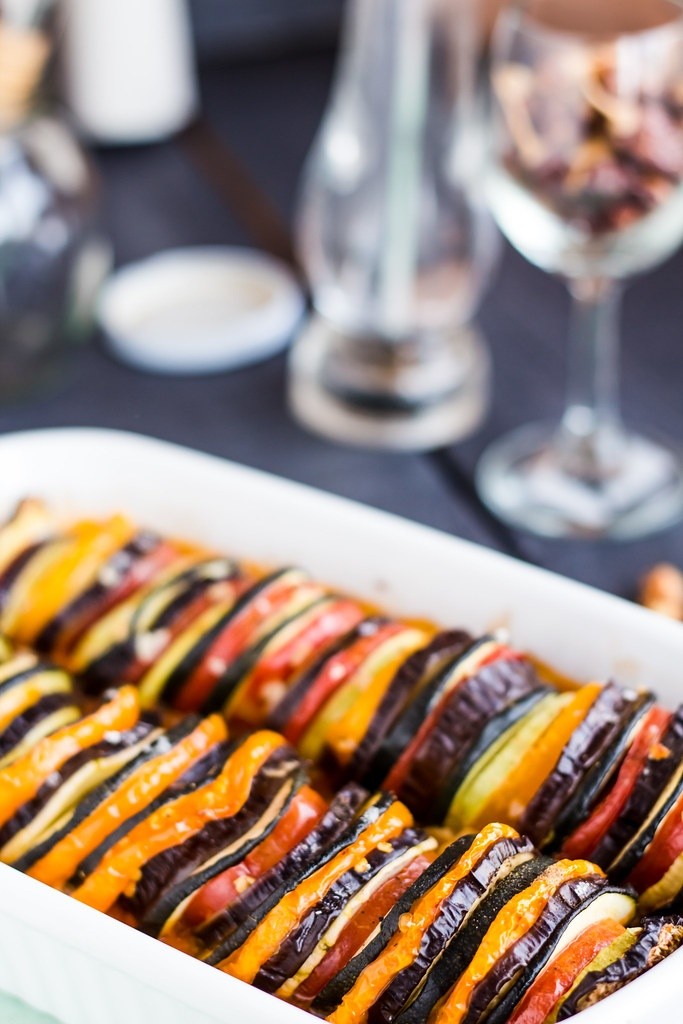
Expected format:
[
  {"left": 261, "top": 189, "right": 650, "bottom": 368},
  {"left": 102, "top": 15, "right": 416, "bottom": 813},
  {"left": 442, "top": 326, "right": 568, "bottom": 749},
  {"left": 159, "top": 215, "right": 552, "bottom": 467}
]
[{"left": 284, "top": 1, "right": 500, "bottom": 452}]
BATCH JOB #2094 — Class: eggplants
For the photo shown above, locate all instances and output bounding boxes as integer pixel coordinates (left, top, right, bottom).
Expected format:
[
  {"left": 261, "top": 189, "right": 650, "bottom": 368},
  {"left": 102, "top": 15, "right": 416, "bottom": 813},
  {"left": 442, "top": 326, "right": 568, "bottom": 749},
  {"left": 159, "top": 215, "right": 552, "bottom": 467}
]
[{"left": 0, "top": 532, "right": 683, "bottom": 1024}]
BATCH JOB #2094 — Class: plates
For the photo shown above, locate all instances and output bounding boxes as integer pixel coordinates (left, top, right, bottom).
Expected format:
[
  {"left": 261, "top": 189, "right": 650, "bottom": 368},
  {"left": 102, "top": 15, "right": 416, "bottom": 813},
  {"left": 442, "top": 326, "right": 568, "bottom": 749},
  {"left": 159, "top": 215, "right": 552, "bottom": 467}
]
[
  {"left": 1, "top": 427, "right": 682, "bottom": 1023},
  {"left": 98, "top": 245, "right": 301, "bottom": 382}
]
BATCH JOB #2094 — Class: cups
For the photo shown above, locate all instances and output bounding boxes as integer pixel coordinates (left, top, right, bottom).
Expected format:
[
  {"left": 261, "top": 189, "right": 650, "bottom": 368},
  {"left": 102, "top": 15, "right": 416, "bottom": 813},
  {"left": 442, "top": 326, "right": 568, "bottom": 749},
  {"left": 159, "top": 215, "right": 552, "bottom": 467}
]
[{"left": 53, "top": 1, "right": 197, "bottom": 151}]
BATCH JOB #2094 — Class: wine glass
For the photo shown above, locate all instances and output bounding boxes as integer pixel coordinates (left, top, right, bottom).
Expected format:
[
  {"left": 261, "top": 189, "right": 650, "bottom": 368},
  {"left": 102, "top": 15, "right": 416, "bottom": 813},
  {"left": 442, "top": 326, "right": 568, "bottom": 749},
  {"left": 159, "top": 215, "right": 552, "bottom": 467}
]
[{"left": 474, "top": 7, "right": 683, "bottom": 537}]
